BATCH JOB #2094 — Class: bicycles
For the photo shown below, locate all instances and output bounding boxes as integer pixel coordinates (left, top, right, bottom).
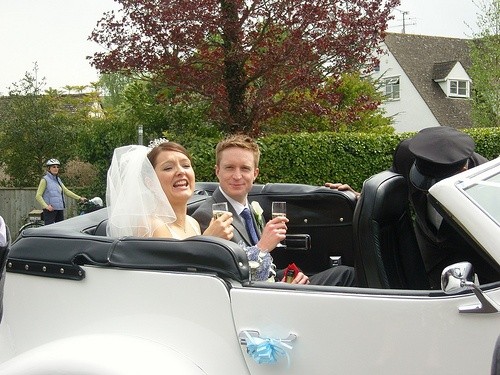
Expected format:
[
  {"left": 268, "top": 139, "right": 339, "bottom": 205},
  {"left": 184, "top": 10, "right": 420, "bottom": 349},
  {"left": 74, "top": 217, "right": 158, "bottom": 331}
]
[{"left": 18, "top": 197, "right": 104, "bottom": 236}]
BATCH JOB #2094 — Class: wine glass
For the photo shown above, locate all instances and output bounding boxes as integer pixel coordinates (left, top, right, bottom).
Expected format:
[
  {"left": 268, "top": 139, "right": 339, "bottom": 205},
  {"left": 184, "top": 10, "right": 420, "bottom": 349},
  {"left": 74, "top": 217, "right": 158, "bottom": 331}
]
[{"left": 272, "top": 202, "right": 287, "bottom": 248}]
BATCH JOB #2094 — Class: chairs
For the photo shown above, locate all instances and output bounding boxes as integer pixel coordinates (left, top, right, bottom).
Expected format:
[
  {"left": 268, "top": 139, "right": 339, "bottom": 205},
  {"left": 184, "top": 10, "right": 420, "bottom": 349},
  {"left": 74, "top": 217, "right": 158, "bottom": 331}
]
[{"left": 355, "top": 170, "right": 415, "bottom": 289}]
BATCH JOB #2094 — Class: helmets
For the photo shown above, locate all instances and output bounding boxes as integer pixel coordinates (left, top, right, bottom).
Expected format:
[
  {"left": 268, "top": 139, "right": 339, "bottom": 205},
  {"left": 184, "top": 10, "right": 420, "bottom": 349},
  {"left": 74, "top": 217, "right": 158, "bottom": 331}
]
[{"left": 47, "top": 158, "right": 60, "bottom": 165}]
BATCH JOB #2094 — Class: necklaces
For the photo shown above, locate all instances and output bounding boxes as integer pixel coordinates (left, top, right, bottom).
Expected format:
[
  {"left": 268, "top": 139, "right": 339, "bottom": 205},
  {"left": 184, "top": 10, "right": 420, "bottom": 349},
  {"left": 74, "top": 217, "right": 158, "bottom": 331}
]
[{"left": 175, "top": 222, "right": 187, "bottom": 233}]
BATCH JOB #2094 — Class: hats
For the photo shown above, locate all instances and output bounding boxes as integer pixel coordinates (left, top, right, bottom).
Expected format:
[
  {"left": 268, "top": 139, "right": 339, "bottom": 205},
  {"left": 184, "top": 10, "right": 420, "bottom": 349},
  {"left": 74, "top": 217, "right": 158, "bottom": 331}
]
[{"left": 408, "top": 126, "right": 474, "bottom": 191}]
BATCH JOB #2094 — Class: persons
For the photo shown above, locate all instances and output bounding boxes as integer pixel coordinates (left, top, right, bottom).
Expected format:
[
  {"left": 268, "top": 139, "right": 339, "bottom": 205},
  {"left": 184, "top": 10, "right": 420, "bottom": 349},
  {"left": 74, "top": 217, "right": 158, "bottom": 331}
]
[
  {"left": 140, "top": 142, "right": 311, "bottom": 286},
  {"left": 190, "top": 136, "right": 359, "bottom": 290},
  {"left": 325, "top": 126, "right": 500, "bottom": 291},
  {"left": 35, "top": 159, "right": 87, "bottom": 225}
]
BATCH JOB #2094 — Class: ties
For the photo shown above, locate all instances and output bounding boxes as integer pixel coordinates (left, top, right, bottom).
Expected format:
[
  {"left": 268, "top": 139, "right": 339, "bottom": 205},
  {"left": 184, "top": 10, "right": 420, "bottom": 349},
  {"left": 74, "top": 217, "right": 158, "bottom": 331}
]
[{"left": 240, "top": 207, "right": 258, "bottom": 245}]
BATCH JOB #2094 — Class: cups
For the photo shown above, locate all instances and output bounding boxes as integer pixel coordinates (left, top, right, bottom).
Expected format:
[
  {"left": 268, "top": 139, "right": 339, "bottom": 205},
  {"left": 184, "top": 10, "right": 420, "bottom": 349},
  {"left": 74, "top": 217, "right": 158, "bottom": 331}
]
[{"left": 212, "top": 202, "right": 228, "bottom": 221}]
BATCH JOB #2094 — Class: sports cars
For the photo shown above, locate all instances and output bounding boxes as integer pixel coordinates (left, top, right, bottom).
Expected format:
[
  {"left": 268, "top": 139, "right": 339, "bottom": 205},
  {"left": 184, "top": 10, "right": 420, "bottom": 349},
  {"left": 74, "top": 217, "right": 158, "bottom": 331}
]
[{"left": 0, "top": 150, "right": 499, "bottom": 375}]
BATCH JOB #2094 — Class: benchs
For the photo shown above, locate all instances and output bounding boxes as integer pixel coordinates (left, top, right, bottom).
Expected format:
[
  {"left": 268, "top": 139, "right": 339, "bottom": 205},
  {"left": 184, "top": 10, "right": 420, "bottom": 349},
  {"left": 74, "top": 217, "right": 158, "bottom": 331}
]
[{"left": 94, "top": 194, "right": 211, "bottom": 235}]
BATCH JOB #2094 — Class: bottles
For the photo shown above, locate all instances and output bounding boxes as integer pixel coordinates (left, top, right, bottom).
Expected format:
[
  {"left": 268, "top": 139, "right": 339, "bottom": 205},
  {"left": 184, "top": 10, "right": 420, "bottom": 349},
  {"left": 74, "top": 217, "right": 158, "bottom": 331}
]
[{"left": 286, "top": 270, "right": 295, "bottom": 284}]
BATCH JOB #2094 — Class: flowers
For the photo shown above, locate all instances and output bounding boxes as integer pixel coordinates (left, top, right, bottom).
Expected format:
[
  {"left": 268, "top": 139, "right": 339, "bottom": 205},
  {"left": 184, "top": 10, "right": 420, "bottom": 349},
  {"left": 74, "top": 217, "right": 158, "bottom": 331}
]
[
  {"left": 240, "top": 241, "right": 277, "bottom": 281},
  {"left": 251, "top": 200, "right": 264, "bottom": 225}
]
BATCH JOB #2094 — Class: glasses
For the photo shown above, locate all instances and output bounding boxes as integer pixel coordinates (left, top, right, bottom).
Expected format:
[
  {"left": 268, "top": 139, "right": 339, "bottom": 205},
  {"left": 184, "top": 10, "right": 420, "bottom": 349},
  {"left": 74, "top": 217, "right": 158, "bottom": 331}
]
[{"left": 53, "top": 166, "right": 60, "bottom": 167}]
